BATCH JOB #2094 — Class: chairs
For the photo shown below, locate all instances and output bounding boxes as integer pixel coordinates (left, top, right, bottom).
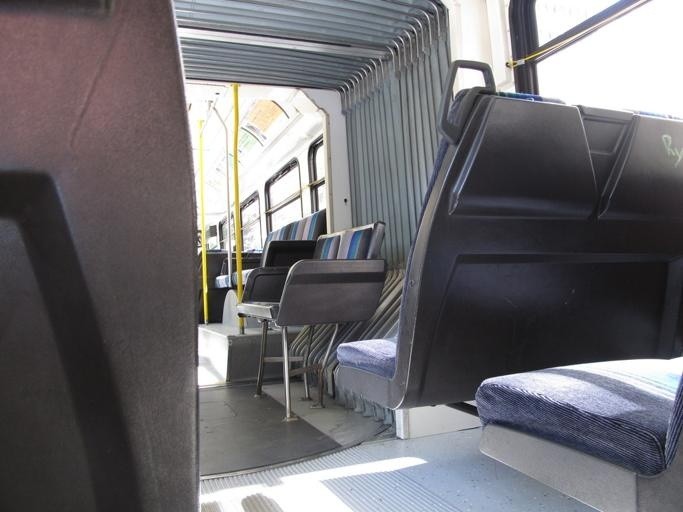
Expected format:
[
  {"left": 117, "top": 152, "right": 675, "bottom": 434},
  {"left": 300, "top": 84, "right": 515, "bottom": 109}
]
[{"left": 0, "top": 0, "right": 199, "bottom": 512}]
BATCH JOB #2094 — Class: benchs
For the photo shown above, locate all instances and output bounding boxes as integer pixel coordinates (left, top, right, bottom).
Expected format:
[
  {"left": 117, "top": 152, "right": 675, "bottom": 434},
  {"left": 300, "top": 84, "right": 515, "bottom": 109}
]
[
  {"left": 214, "top": 209, "right": 326, "bottom": 287},
  {"left": 198, "top": 249, "right": 262, "bottom": 321},
  {"left": 333, "top": 59, "right": 683, "bottom": 443},
  {"left": 471, "top": 355, "right": 682, "bottom": 511},
  {"left": 234, "top": 221, "right": 389, "bottom": 422}
]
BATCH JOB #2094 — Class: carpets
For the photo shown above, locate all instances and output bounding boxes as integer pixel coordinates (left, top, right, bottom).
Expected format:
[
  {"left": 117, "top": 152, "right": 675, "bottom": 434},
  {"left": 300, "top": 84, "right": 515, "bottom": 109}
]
[{"left": 197, "top": 376, "right": 341, "bottom": 479}]
